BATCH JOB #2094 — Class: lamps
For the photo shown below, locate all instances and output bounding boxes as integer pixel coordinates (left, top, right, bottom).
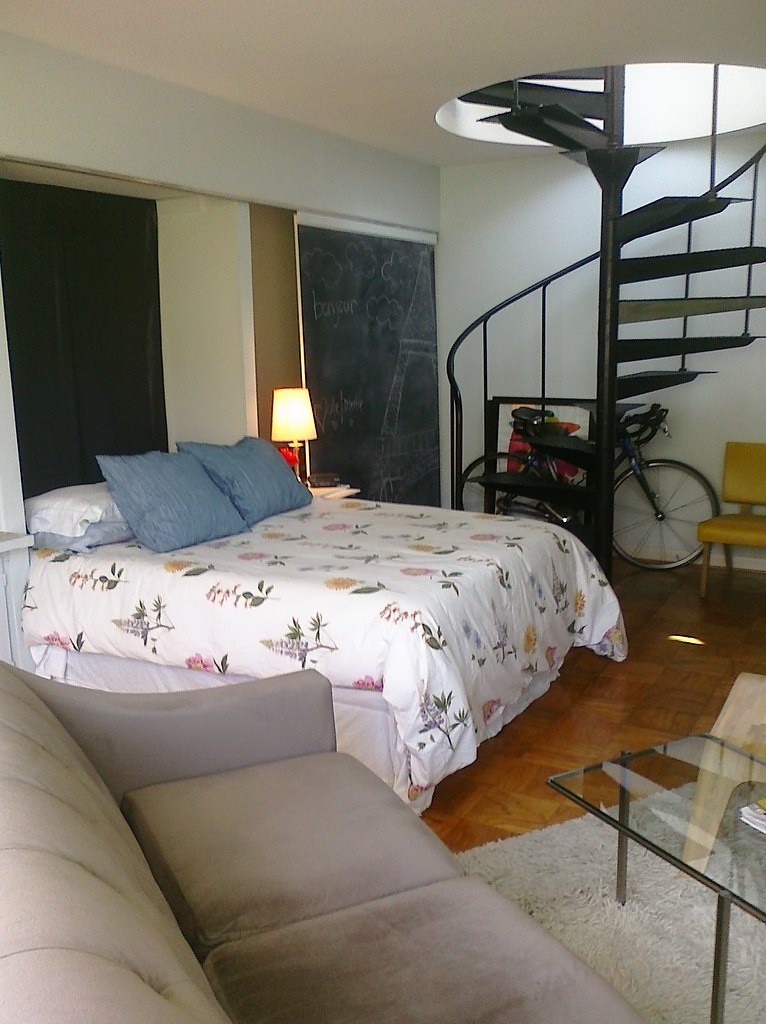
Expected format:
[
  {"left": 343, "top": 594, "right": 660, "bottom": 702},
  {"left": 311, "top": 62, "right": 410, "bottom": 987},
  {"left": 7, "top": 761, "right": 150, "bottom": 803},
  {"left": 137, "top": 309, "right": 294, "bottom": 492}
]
[{"left": 271, "top": 388, "right": 317, "bottom": 481}]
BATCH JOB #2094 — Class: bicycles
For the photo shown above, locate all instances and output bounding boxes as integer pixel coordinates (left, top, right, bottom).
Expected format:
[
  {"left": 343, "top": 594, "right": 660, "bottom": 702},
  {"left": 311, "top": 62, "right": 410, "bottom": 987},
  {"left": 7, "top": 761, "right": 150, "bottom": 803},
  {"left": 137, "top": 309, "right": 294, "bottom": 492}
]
[{"left": 459, "top": 404, "right": 719, "bottom": 573}]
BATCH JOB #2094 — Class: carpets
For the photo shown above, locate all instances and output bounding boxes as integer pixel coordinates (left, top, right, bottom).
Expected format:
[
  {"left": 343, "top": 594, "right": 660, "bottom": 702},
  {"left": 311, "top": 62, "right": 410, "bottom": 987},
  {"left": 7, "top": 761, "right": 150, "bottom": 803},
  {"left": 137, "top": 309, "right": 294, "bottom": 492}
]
[{"left": 454, "top": 779, "right": 766, "bottom": 1024}]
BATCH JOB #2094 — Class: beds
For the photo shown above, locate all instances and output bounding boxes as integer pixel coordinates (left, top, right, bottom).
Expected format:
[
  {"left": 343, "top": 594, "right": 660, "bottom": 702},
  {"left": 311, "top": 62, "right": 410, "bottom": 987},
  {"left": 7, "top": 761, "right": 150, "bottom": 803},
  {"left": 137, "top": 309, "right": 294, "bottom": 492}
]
[{"left": 19, "top": 435, "right": 630, "bottom": 818}]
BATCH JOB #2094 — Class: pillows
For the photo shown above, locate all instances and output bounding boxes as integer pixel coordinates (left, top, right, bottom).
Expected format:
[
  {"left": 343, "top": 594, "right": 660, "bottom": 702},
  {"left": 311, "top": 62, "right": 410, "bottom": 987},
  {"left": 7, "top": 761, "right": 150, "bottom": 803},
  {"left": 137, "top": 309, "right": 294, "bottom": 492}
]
[
  {"left": 25, "top": 481, "right": 127, "bottom": 538},
  {"left": 175, "top": 436, "right": 314, "bottom": 528},
  {"left": 32, "top": 519, "right": 135, "bottom": 557},
  {"left": 94, "top": 451, "right": 251, "bottom": 551}
]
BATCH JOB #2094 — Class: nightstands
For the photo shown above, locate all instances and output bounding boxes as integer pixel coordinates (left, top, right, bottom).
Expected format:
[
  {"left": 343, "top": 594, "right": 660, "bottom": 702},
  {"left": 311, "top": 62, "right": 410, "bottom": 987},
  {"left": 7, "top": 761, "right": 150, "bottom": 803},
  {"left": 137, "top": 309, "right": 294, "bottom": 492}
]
[
  {"left": 0, "top": 530, "right": 35, "bottom": 670},
  {"left": 302, "top": 479, "right": 362, "bottom": 499}
]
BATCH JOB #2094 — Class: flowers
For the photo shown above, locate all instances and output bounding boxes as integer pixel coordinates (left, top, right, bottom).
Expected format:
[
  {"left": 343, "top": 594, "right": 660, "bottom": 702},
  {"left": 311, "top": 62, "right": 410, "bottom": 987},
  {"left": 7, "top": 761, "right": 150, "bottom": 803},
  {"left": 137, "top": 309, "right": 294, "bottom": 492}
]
[{"left": 280, "top": 447, "right": 300, "bottom": 468}]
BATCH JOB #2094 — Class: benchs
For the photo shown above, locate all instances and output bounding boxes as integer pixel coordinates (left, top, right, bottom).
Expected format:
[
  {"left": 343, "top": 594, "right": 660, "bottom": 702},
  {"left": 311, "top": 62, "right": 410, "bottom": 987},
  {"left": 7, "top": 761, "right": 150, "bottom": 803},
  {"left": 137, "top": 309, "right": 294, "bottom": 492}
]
[{"left": 696, "top": 441, "right": 766, "bottom": 598}]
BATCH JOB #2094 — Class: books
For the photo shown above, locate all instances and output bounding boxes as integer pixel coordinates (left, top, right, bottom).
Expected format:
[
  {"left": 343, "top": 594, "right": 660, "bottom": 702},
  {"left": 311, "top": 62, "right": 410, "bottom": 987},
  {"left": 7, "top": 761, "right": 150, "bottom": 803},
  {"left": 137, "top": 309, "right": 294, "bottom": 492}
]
[{"left": 739, "top": 796, "right": 766, "bottom": 835}]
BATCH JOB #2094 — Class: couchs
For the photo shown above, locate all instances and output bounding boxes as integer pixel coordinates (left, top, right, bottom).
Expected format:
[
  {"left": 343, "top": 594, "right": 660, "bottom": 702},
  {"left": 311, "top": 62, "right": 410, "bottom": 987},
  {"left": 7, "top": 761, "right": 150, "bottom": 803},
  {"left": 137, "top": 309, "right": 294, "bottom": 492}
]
[{"left": 0, "top": 659, "right": 647, "bottom": 1024}]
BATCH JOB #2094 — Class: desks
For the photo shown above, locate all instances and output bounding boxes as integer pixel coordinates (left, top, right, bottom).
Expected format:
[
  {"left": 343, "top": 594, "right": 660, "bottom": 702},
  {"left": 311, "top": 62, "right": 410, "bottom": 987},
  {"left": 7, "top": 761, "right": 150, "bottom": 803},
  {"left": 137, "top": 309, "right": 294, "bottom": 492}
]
[{"left": 545, "top": 733, "right": 766, "bottom": 1024}]
